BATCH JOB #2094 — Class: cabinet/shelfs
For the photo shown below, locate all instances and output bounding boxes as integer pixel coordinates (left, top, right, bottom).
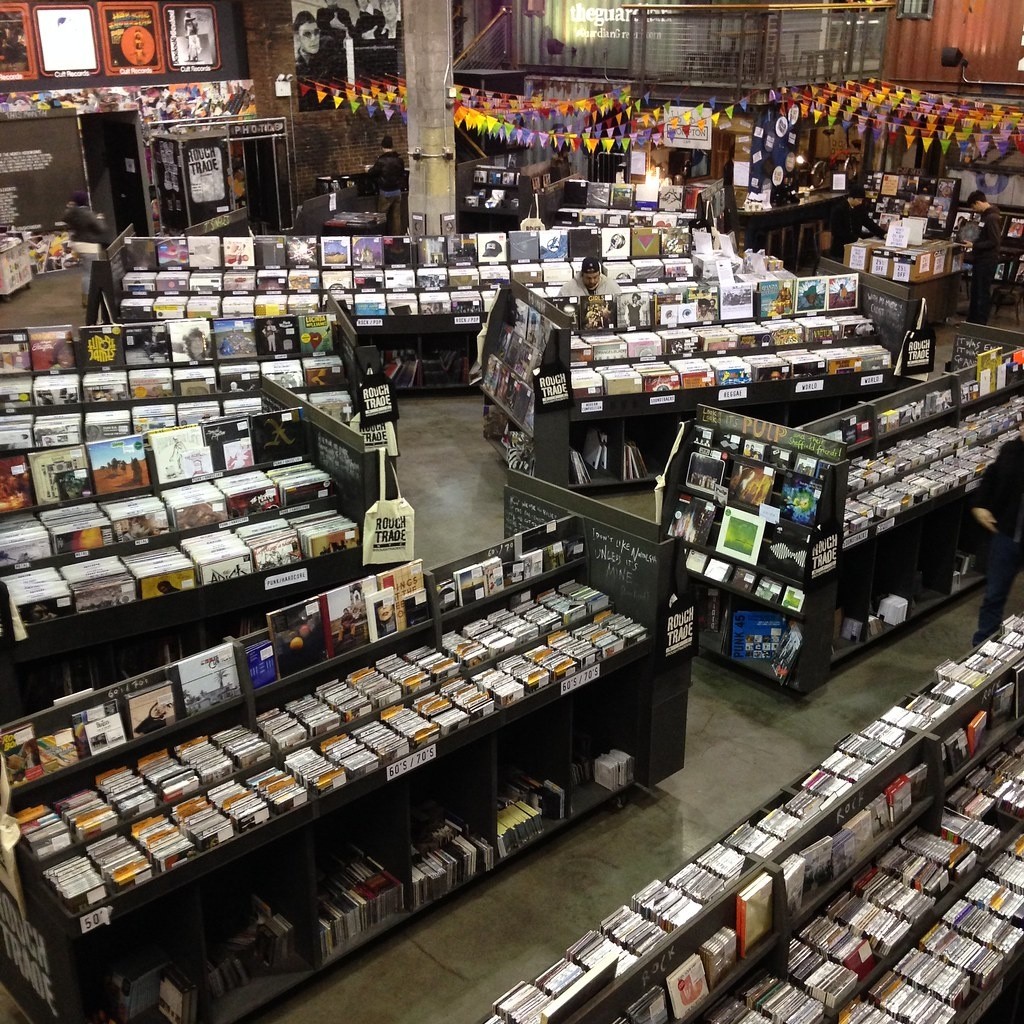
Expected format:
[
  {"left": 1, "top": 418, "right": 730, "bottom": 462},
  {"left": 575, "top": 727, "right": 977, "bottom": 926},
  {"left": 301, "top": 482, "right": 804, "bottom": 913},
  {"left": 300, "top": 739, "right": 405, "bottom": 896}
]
[{"left": 0, "top": 158, "right": 1024, "bottom": 1024}]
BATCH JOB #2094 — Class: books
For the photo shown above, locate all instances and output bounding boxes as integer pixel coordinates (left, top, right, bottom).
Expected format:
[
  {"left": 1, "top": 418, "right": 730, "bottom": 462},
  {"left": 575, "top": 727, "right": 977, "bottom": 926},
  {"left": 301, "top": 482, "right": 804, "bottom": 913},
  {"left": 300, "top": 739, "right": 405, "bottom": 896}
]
[{"left": 0, "top": 164, "right": 1024, "bottom": 1024}]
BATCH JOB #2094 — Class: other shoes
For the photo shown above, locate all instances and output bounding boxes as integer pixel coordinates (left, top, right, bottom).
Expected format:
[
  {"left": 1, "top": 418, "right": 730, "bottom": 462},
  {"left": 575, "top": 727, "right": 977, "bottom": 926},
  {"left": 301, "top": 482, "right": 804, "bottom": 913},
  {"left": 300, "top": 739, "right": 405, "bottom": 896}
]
[{"left": 954, "top": 320, "right": 967, "bottom": 329}]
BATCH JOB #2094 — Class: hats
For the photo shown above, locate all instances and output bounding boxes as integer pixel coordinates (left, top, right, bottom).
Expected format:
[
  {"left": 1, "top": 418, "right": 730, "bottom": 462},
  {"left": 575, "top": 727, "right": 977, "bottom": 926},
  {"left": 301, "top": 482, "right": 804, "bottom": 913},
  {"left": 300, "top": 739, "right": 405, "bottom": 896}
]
[
  {"left": 69, "top": 189, "right": 88, "bottom": 205},
  {"left": 632, "top": 293, "right": 642, "bottom": 301},
  {"left": 581, "top": 257, "right": 600, "bottom": 274},
  {"left": 381, "top": 136, "right": 394, "bottom": 148},
  {"left": 482, "top": 240, "right": 503, "bottom": 257},
  {"left": 847, "top": 184, "right": 866, "bottom": 198}
]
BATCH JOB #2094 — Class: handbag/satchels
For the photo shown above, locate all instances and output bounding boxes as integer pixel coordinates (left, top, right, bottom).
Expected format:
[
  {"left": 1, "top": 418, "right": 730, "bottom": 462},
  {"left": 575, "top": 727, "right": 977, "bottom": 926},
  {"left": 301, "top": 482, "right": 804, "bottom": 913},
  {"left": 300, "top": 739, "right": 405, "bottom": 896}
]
[
  {"left": 362, "top": 446, "right": 416, "bottom": 567},
  {"left": 531, "top": 328, "right": 578, "bottom": 416},
  {"left": 476, "top": 288, "right": 502, "bottom": 367},
  {"left": 893, "top": 298, "right": 929, "bottom": 382},
  {"left": 349, "top": 367, "right": 399, "bottom": 457},
  {"left": 352, "top": 345, "right": 400, "bottom": 430},
  {"left": 705, "top": 200, "right": 718, "bottom": 239},
  {"left": 901, "top": 298, "right": 936, "bottom": 377},
  {"left": 0, "top": 753, "right": 28, "bottom": 922},
  {"left": 519, "top": 193, "right": 546, "bottom": 231},
  {"left": 688, "top": 193, "right": 712, "bottom": 251}
]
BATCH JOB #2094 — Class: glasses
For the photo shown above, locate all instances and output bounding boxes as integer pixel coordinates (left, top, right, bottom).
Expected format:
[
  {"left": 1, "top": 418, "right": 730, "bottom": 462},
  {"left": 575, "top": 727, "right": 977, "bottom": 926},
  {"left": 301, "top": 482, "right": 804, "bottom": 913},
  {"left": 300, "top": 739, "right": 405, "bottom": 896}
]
[{"left": 302, "top": 28, "right": 322, "bottom": 38}]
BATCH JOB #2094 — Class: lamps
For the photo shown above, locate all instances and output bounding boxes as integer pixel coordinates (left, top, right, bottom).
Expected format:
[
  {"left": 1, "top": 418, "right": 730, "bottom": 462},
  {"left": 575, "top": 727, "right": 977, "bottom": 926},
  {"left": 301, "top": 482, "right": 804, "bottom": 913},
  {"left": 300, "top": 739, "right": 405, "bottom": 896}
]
[
  {"left": 941, "top": 46, "right": 969, "bottom": 68},
  {"left": 274, "top": 73, "right": 293, "bottom": 99},
  {"left": 546, "top": 37, "right": 577, "bottom": 56}
]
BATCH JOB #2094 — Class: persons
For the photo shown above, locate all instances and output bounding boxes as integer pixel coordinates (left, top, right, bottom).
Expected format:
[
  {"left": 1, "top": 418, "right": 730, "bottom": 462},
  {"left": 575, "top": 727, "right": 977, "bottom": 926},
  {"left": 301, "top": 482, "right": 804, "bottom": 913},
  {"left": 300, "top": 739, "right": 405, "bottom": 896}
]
[
  {"left": 365, "top": 135, "right": 405, "bottom": 236},
  {"left": 967, "top": 425, "right": 1024, "bottom": 649},
  {"left": 829, "top": 185, "right": 888, "bottom": 264},
  {"left": 557, "top": 256, "right": 622, "bottom": 297},
  {"left": 293, "top": 0, "right": 402, "bottom": 111},
  {"left": 959, "top": 189, "right": 1001, "bottom": 326},
  {"left": 62, "top": 190, "right": 109, "bottom": 243}
]
